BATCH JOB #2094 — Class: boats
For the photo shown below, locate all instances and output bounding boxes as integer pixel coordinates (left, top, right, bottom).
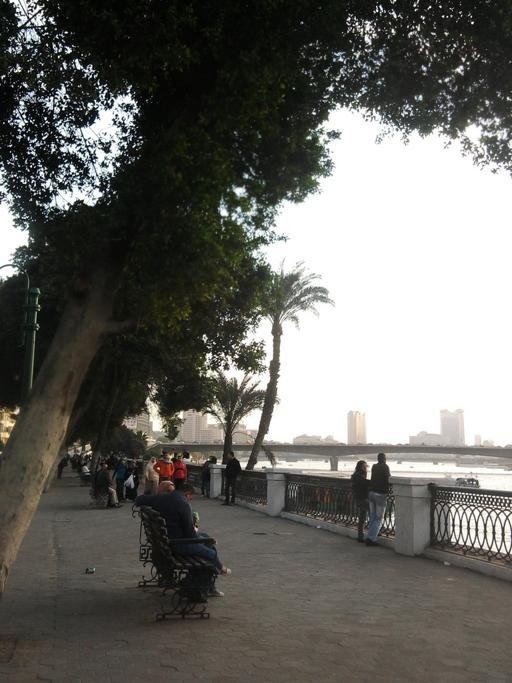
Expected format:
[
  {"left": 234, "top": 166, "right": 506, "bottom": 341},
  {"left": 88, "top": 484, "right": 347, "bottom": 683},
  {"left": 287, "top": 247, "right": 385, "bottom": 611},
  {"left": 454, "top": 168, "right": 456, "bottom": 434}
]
[{"left": 455, "top": 476, "right": 480, "bottom": 490}]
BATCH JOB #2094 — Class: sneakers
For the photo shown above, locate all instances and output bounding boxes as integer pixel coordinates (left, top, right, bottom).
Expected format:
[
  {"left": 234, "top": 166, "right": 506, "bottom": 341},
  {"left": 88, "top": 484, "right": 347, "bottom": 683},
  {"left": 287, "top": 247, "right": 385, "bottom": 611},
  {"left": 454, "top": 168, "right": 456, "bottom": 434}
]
[
  {"left": 209, "top": 589, "right": 224, "bottom": 597},
  {"left": 358, "top": 529, "right": 378, "bottom": 547},
  {"left": 220, "top": 566, "right": 231, "bottom": 576}
]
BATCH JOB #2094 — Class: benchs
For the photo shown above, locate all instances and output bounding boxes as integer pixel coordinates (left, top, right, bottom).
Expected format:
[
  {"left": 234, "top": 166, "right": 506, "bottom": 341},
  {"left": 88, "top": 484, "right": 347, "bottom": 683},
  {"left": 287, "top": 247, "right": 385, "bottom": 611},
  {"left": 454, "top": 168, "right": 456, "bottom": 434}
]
[
  {"left": 131, "top": 492, "right": 221, "bottom": 623},
  {"left": 89, "top": 468, "right": 112, "bottom": 510}
]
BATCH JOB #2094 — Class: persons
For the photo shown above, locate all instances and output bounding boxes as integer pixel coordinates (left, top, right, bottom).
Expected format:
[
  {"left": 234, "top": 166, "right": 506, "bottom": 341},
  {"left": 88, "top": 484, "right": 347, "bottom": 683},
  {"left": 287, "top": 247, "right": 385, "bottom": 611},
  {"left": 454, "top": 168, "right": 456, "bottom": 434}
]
[
  {"left": 366, "top": 451, "right": 391, "bottom": 545},
  {"left": 56, "top": 449, "right": 217, "bottom": 513},
  {"left": 158, "top": 482, "right": 232, "bottom": 599},
  {"left": 349, "top": 459, "right": 371, "bottom": 544},
  {"left": 221, "top": 451, "right": 242, "bottom": 506},
  {"left": 133, "top": 479, "right": 218, "bottom": 555}
]
